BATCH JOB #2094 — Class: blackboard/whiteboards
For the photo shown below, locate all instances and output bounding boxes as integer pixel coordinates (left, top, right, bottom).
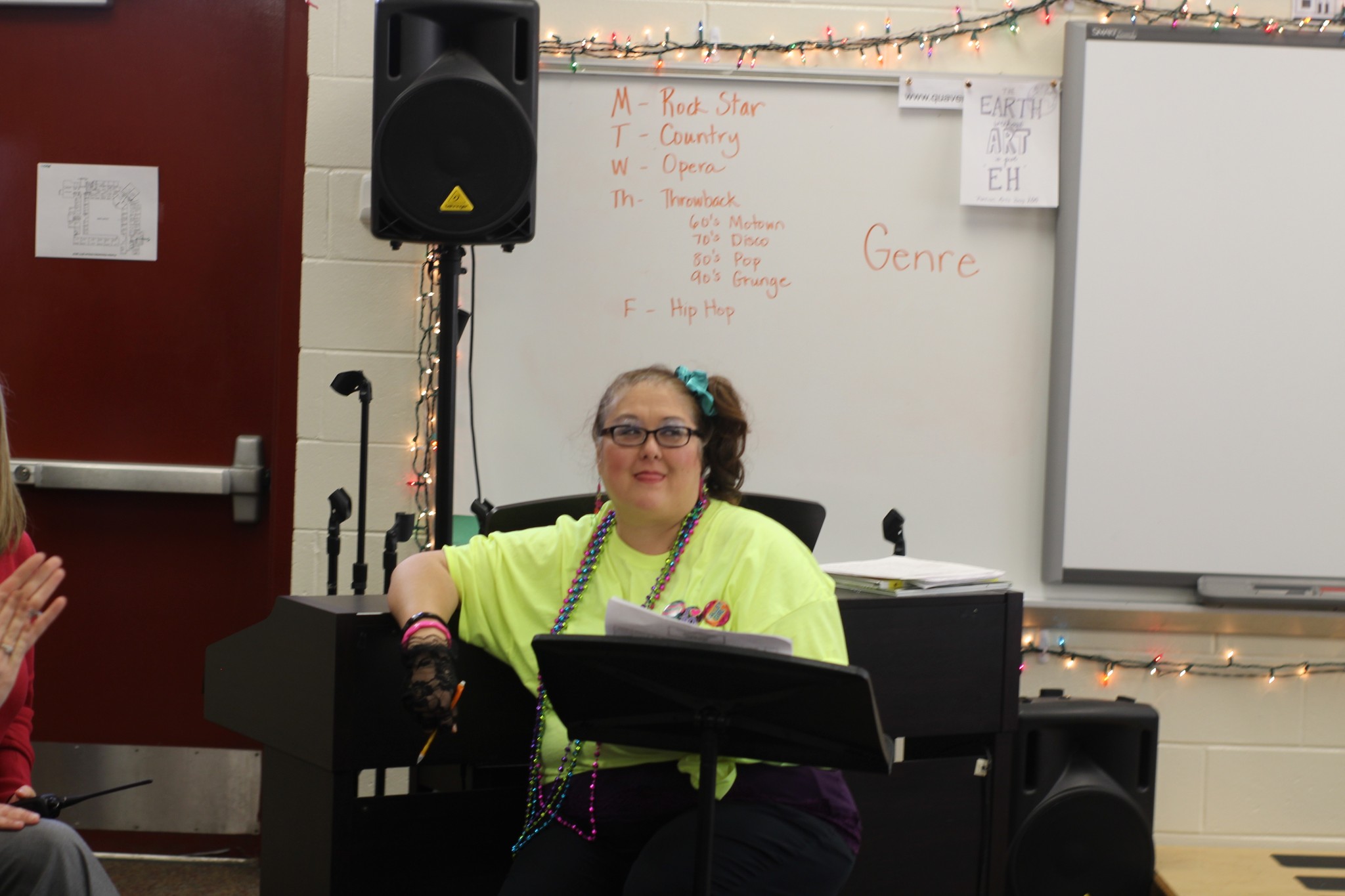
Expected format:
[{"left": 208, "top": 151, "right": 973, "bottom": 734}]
[
  {"left": 452, "top": 58, "right": 1345, "bottom": 602},
  {"left": 1043, "top": 21, "right": 1345, "bottom": 600}
]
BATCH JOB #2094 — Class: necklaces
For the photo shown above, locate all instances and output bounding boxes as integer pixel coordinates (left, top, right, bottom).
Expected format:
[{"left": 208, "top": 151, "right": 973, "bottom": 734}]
[{"left": 512, "top": 491, "right": 712, "bottom": 855}]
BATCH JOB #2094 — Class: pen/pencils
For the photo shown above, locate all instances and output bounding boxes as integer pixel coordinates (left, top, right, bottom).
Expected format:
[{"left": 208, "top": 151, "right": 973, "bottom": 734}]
[{"left": 416, "top": 681, "right": 465, "bottom": 765}]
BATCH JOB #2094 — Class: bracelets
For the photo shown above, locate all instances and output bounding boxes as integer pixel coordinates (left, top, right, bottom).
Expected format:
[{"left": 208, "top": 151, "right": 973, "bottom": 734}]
[{"left": 399, "top": 609, "right": 450, "bottom": 648}]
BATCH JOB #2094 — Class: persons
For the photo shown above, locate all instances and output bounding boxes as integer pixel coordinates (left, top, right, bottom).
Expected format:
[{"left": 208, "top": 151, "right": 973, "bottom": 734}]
[
  {"left": 0, "top": 381, "right": 123, "bottom": 896},
  {"left": 0, "top": 553, "right": 67, "bottom": 710},
  {"left": 385, "top": 364, "right": 868, "bottom": 896}
]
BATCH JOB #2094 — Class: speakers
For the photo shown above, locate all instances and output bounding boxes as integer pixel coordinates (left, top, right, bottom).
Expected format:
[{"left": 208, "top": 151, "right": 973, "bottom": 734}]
[
  {"left": 370, "top": 0, "right": 540, "bottom": 243},
  {"left": 830, "top": 581, "right": 1024, "bottom": 896},
  {"left": 1014, "top": 694, "right": 1159, "bottom": 896}
]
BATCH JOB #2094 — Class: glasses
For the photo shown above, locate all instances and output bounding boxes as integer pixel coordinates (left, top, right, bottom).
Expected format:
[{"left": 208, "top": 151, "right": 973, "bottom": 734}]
[{"left": 600, "top": 424, "right": 702, "bottom": 448}]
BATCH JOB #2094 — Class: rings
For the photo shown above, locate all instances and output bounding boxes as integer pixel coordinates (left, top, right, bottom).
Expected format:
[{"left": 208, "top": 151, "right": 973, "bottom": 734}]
[{"left": 1, "top": 644, "right": 13, "bottom": 655}]
